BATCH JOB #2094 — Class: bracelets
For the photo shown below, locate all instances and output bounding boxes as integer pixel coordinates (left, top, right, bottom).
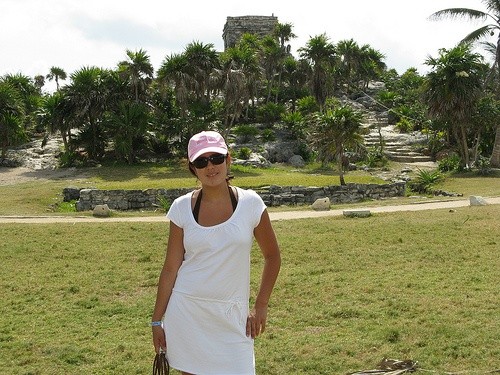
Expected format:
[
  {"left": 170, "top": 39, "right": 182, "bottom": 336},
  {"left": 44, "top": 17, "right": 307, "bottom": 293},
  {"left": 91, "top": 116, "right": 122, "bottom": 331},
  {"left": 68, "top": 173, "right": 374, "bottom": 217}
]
[{"left": 151, "top": 320, "right": 161, "bottom": 327}]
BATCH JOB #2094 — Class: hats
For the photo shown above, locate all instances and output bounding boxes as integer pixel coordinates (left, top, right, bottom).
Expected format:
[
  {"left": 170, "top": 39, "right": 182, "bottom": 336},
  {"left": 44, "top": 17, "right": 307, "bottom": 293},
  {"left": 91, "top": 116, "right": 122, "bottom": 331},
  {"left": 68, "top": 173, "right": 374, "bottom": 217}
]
[{"left": 187, "top": 130, "right": 228, "bottom": 163}]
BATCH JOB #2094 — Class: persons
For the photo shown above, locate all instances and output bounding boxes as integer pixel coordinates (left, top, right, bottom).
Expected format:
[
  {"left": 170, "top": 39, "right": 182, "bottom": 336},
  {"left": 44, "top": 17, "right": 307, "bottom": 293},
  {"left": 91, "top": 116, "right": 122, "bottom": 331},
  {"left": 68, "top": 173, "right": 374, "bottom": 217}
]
[{"left": 151, "top": 131, "right": 281, "bottom": 375}]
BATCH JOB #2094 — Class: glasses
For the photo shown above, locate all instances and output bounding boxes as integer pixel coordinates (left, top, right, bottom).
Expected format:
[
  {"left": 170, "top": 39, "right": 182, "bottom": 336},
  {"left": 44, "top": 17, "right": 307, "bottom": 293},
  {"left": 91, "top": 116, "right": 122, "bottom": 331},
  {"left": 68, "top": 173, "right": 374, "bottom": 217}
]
[{"left": 191, "top": 153, "right": 227, "bottom": 169}]
